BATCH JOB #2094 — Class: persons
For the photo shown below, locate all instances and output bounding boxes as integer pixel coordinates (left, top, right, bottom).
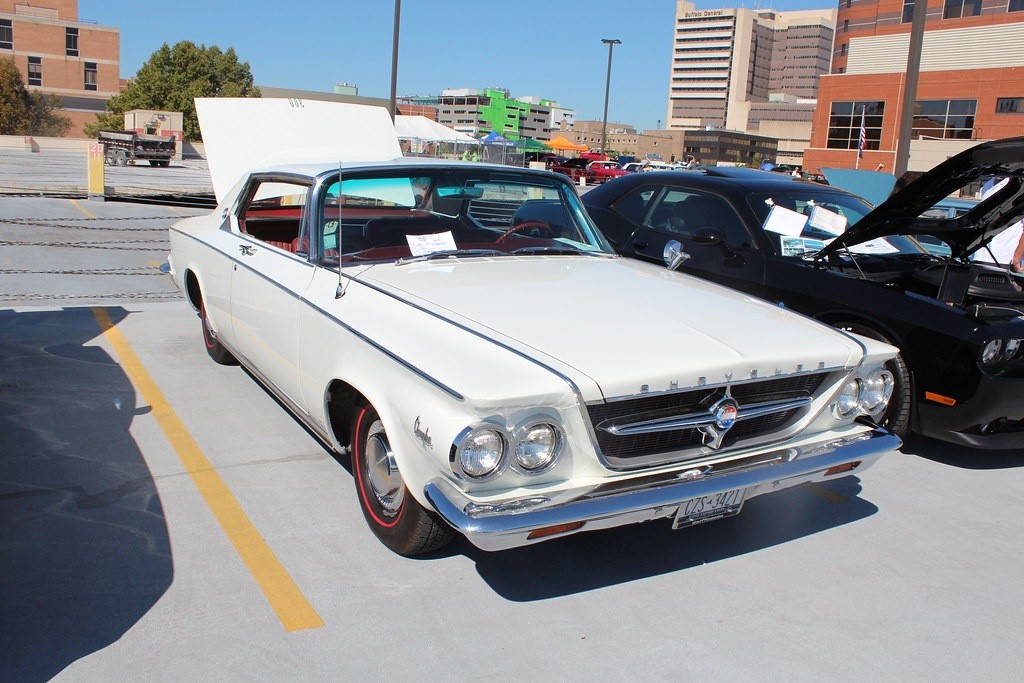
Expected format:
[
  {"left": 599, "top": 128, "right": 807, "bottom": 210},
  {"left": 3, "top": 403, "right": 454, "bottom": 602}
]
[
  {"left": 972, "top": 176, "right": 1024, "bottom": 274},
  {"left": 462, "top": 148, "right": 478, "bottom": 162},
  {"left": 783, "top": 166, "right": 802, "bottom": 178}
]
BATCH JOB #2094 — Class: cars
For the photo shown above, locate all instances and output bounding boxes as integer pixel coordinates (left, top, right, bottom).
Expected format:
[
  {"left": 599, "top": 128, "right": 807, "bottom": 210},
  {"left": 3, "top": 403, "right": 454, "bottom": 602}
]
[
  {"left": 493, "top": 148, "right": 687, "bottom": 184},
  {"left": 510, "top": 136, "right": 1024, "bottom": 461},
  {"left": 159, "top": 97, "right": 904, "bottom": 559}
]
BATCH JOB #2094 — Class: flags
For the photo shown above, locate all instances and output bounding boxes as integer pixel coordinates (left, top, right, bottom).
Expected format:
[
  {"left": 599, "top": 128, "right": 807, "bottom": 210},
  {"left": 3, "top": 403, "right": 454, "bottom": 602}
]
[{"left": 858, "top": 105, "right": 867, "bottom": 158}]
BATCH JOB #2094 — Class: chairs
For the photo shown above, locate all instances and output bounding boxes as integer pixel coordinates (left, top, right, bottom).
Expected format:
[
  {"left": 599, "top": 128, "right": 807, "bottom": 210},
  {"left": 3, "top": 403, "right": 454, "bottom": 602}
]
[
  {"left": 365, "top": 218, "right": 445, "bottom": 248},
  {"left": 454, "top": 229, "right": 500, "bottom": 245},
  {"left": 615, "top": 194, "right": 664, "bottom": 227}
]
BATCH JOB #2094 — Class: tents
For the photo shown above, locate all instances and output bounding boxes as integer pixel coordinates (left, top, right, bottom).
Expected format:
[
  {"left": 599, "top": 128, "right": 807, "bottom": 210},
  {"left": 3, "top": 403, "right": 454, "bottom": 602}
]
[
  {"left": 394, "top": 115, "right": 479, "bottom": 159},
  {"left": 476, "top": 130, "right": 588, "bottom": 168}
]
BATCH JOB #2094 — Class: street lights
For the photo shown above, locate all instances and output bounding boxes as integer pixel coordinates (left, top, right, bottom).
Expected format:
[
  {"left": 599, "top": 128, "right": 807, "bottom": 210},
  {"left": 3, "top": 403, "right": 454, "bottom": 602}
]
[{"left": 600, "top": 39, "right": 622, "bottom": 168}]
